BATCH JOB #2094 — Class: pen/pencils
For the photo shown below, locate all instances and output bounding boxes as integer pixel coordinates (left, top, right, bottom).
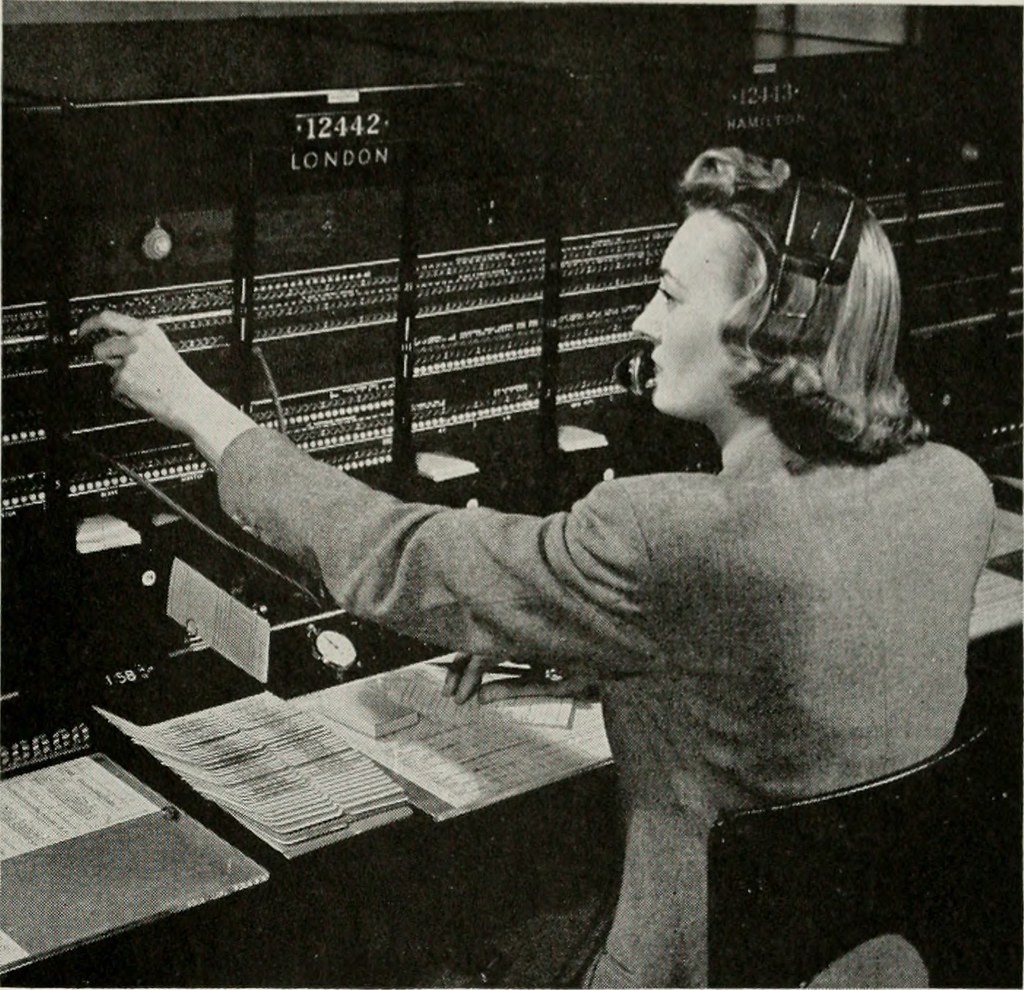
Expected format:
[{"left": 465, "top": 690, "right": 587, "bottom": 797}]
[{"left": 426, "top": 658, "right": 532, "bottom": 675}]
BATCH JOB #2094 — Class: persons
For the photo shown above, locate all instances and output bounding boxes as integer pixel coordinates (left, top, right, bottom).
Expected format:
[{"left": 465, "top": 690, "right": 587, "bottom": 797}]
[{"left": 69, "top": 146, "right": 997, "bottom": 989}]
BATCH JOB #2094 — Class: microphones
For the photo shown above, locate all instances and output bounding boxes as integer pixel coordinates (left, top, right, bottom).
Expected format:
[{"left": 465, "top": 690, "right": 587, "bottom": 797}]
[{"left": 613, "top": 348, "right": 656, "bottom": 395}]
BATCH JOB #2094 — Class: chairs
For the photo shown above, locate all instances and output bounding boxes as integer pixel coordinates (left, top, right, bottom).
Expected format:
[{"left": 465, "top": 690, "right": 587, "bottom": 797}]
[{"left": 706, "top": 662, "right": 1022, "bottom": 988}]
